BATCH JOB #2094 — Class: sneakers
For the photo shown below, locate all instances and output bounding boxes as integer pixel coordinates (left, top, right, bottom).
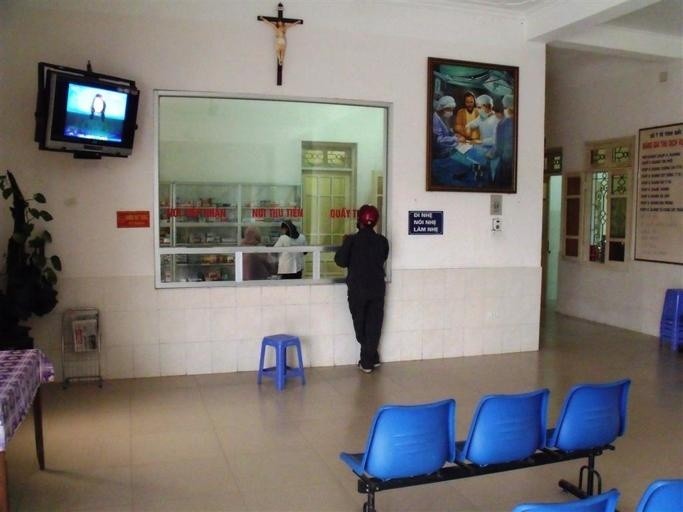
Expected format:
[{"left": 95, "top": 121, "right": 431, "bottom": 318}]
[{"left": 358, "top": 360, "right": 382, "bottom": 373}]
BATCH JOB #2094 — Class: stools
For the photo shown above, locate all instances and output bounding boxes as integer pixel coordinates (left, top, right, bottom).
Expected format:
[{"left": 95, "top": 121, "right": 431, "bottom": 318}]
[{"left": 660, "top": 288, "right": 683, "bottom": 353}]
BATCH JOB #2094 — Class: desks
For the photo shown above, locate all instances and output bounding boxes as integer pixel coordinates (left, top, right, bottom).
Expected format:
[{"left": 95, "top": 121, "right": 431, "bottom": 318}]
[{"left": 0, "top": 350, "right": 56, "bottom": 511}]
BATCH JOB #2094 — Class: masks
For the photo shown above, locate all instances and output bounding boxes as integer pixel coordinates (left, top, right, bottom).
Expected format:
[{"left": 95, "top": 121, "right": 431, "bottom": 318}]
[
  {"left": 478, "top": 110, "right": 489, "bottom": 120},
  {"left": 443, "top": 110, "right": 453, "bottom": 119},
  {"left": 503, "top": 109, "right": 512, "bottom": 119}
]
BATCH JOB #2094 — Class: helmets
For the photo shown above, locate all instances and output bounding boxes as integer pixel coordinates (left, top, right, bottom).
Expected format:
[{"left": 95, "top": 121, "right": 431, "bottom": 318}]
[{"left": 358, "top": 205, "right": 379, "bottom": 227}]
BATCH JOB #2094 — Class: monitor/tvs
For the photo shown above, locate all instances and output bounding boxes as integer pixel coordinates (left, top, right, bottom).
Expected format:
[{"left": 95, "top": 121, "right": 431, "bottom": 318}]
[{"left": 39, "top": 69, "right": 140, "bottom": 157}]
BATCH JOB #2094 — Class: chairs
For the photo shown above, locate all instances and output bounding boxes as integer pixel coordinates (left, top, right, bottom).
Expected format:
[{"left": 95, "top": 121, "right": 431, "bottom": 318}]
[
  {"left": 457, "top": 387, "right": 547, "bottom": 477},
  {"left": 635, "top": 478, "right": 683, "bottom": 512},
  {"left": 512, "top": 488, "right": 621, "bottom": 512},
  {"left": 547, "top": 378, "right": 630, "bottom": 500},
  {"left": 257, "top": 334, "right": 307, "bottom": 388},
  {"left": 339, "top": 399, "right": 456, "bottom": 512}
]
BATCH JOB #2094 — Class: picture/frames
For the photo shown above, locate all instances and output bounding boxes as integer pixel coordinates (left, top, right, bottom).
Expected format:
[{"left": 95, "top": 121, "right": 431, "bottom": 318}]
[{"left": 426, "top": 56, "right": 520, "bottom": 194}]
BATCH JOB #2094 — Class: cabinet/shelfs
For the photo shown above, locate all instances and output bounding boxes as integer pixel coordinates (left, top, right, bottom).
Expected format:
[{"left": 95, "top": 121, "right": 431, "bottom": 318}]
[{"left": 159, "top": 180, "right": 303, "bottom": 281}]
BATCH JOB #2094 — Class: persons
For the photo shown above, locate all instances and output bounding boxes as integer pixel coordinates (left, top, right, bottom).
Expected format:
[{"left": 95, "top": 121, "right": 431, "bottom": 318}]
[
  {"left": 271, "top": 218, "right": 308, "bottom": 279},
  {"left": 454, "top": 91, "right": 480, "bottom": 140},
  {"left": 466, "top": 94, "right": 500, "bottom": 182},
  {"left": 494, "top": 94, "right": 513, "bottom": 186},
  {"left": 89, "top": 93, "right": 107, "bottom": 122},
  {"left": 332, "top": 204, "right": 391, "bottom": 373},
  {"left": 240, "top": 225, "right": 270, "bottom": 280},
  {"left": 431, "top": 96, "right": 467, "bottom": 158}
]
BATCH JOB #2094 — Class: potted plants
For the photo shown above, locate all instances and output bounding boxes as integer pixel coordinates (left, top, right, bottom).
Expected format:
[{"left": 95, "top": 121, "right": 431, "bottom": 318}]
[{"left": 0, "top": 171, "right": 61, "bottom": 351}]
